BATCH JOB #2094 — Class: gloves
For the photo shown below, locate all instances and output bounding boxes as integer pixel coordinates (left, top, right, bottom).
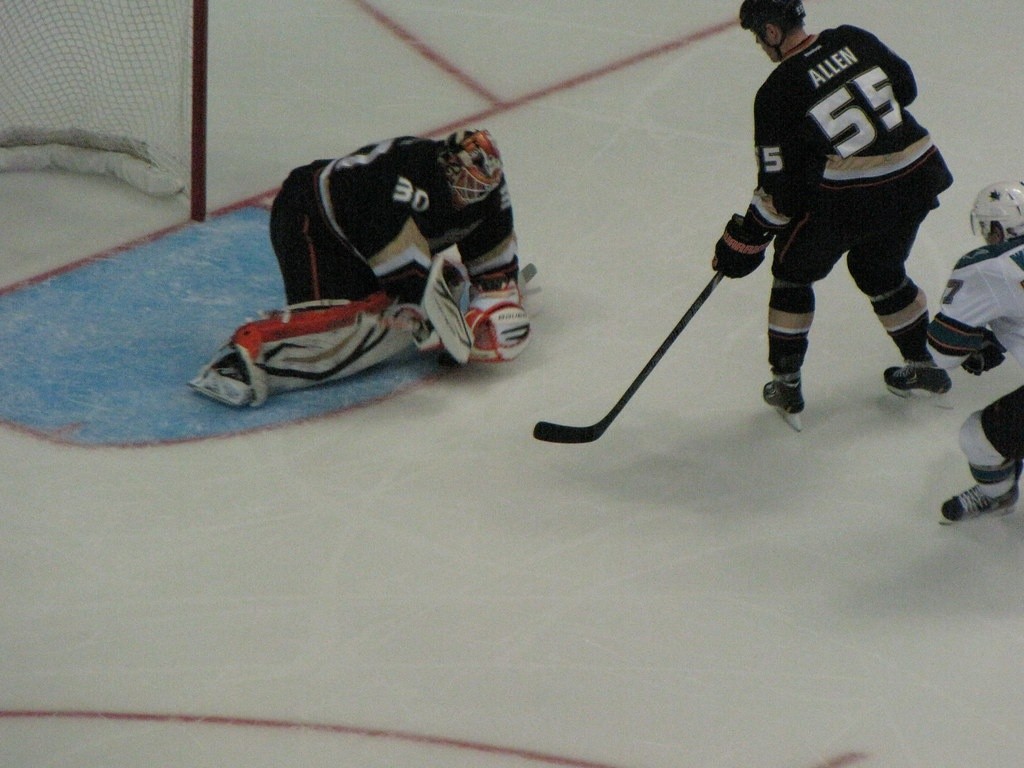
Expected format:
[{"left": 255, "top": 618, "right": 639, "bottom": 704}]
[
  {"left": 977, "top": 326, "right": 1007, "bottom": 353},
  {"left": 961, "top": 345, "right": 1006, "bottom": 375},
  {"left": 712, "top": 212, "right": 774, "bottom": 279}
]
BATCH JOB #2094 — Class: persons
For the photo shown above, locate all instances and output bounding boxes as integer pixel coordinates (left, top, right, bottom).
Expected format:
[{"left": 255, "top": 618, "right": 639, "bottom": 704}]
[
  {"left": 188, "top": 129, "right": 530, "bottom": 410},
  {"left": 712, "top": 0, "right": 953, "bottom": 431},
  {"left": 924, "top": 180, "right": 1024, "bottom": 526}
]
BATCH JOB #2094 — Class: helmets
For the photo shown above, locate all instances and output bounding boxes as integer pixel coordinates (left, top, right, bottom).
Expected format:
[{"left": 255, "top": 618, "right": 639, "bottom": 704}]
[
  {"left": 739, "top": 0, "right": 807, "bottom": 30},
  {"left": 437, "top": 129, "right": 502, "bottom": 207},
  {"left": 970, "top": 181, "right": 1024, "bottom": 242}
]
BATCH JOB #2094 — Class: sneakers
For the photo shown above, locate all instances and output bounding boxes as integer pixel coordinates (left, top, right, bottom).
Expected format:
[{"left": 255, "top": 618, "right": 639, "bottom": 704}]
[
  {"left": 764, "top": 369, "right": 804, "bottom": 431},
  {"left": 938, "top": 459, "right": 1024, "bottom": 524},
  {"left": 884, "top": 365, "right": 952, "bottom": 398},
  {"left": 187, "top": 342, "right": 252, "bottom": 407}
]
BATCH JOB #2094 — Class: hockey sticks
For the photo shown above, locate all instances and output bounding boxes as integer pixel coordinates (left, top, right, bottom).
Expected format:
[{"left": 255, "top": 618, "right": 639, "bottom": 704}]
[{"left": 532, "top": 270, "right": 723, "bottom": 446}]
